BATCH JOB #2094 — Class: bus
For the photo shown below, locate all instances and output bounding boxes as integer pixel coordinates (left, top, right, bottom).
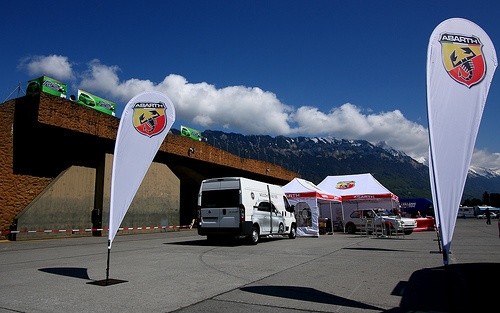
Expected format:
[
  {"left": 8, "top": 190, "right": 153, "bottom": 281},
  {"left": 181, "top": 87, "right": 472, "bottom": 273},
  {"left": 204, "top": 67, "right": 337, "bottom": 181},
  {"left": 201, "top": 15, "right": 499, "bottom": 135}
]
[{"left": 457, "top": 207, "right": 475, "bottom": 217}]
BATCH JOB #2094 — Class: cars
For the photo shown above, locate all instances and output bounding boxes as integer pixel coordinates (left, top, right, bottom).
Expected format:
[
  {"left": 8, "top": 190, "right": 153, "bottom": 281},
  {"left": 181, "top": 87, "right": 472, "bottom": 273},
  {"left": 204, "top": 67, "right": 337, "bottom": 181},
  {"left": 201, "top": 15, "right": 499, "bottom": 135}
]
[{"left": 477, "top": 212, "right": 496, "bottom": 219}]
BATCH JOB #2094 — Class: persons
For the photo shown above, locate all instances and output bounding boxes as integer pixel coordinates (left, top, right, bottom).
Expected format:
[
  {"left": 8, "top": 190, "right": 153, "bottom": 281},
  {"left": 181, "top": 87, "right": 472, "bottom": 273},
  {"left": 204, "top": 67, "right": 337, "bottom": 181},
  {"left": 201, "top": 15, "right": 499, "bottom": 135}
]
[{"left": 485, "top": 208, "right": 493, "bottom": 225}]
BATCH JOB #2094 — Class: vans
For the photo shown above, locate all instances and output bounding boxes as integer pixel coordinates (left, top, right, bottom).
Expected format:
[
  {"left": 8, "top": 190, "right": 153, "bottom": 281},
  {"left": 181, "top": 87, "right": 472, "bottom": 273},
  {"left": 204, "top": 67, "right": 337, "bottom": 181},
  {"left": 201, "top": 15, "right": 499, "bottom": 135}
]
[{"left": 197, "top": 177, "right": 297, "bottom": 245}]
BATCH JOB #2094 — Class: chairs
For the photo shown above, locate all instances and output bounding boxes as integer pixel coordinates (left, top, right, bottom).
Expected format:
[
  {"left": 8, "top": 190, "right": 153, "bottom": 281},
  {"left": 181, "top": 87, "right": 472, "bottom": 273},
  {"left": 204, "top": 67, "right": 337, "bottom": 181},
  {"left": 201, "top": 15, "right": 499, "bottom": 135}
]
[{"left": 357, "top": 217, "right": 405, "bottom": 239}]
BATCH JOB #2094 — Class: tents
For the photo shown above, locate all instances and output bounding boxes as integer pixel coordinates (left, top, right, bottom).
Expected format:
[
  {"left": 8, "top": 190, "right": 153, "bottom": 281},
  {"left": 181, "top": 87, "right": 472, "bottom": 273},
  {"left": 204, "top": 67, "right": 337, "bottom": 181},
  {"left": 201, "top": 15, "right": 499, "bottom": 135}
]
[
  {"left": 282, "top": 178, "right": 345, "bottom": 237},
  {"left": 317, "top": 173, "right": 401, "bottom": 238}
]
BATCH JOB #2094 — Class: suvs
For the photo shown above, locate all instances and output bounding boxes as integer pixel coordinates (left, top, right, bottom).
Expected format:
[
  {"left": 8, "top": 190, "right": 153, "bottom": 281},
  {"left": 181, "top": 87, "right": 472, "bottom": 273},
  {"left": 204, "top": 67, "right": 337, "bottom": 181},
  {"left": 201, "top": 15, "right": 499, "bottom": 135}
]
[{"left": 345, "top": 209, "right": 415, "bottom": 235}]
